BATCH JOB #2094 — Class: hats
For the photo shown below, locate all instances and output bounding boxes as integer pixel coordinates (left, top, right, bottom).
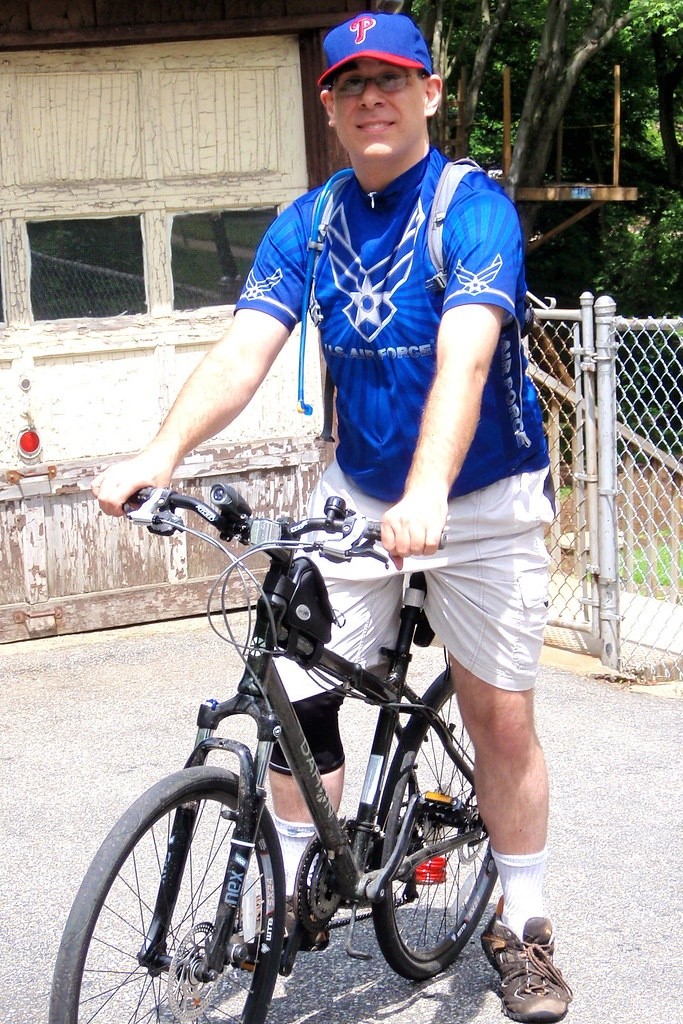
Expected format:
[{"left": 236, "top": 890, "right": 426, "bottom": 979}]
[{"left": 317, "top": 13, "right": 432, "bottom": 89}]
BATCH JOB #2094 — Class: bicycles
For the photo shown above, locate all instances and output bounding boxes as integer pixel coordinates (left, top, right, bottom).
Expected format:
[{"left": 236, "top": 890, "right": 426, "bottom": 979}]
[{"left": 46, "top": 481, "right": 500, "bottom": 1024}]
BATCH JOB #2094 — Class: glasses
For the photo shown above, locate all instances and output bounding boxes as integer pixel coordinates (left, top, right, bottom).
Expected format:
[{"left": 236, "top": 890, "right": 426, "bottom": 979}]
[{"left": 330, "top": 71, "right": 424, "bottom": 97}]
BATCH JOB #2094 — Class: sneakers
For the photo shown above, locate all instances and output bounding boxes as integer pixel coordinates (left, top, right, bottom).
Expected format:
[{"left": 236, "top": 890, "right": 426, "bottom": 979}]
[
  {"left": 482, "top": 896, "right": 574, "bottom": 1022},
  {"left": 279, "top": 896, "right": 328, "bottom": 954}
]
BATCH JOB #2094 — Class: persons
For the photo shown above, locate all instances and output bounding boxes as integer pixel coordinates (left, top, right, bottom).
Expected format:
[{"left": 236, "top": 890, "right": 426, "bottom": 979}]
[{"left": 89, "top": 12, "right": 571, "bottom": 1022}]
